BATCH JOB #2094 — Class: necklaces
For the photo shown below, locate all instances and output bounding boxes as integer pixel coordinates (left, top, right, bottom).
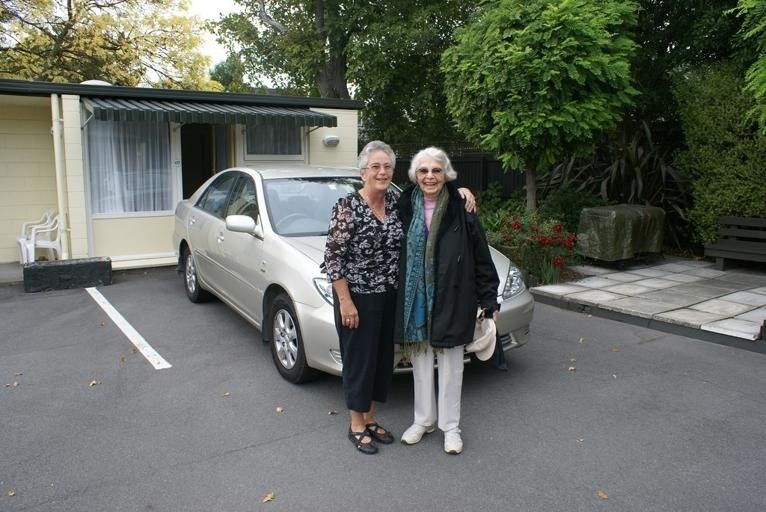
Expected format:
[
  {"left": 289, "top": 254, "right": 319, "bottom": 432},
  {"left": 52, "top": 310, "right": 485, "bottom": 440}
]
[{"left": 363, "top": 184, "right": 387, "bottom": 222}]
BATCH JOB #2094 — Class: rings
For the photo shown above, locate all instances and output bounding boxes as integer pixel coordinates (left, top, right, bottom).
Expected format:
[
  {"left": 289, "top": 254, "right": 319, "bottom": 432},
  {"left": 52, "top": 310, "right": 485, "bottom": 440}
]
[
  {"left": 346, "top": 318, "right": 350, "bottom": 322},
  {"left": 473, "top": 200, "right": 476, "bottom": 204}
]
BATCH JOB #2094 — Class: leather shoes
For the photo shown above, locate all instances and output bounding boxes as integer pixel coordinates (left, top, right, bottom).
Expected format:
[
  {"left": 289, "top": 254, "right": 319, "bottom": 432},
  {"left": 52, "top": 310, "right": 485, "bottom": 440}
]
[
  {"left": 348, "top": 423, "right": 377, "bottom": 454},
  {"left": 366, "top": 423, "right": 393, "bottom": 444}
]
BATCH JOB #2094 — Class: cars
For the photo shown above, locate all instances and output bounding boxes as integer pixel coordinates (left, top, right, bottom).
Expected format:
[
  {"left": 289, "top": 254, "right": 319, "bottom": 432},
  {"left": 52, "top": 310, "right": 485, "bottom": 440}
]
[{"left": 173, "top": 166, "right": 534, "bottom": 385}]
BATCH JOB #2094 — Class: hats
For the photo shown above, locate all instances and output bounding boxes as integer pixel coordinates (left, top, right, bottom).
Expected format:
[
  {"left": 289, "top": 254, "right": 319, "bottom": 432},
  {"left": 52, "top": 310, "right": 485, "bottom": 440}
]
[{"left": 466, "top": 317, "right": 497, "bottom": 361}]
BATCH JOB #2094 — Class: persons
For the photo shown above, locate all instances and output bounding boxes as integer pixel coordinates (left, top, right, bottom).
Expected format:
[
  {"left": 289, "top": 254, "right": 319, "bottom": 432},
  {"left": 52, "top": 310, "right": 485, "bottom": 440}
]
[
  {"left": 323, "top": 140, "right": 478, "bottom": 454},
  {"left": 395, "top": 144, "right": 501, "bottom": 454}
]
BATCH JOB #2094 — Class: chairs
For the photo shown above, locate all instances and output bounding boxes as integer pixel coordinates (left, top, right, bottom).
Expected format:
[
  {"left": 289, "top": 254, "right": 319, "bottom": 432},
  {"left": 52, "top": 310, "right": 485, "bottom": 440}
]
[
  {"left": 24, "top": 211, "right": 62, "bottom": 263},
  {"left": 15, "top": 209, "right": 54, "bottom": 265}
]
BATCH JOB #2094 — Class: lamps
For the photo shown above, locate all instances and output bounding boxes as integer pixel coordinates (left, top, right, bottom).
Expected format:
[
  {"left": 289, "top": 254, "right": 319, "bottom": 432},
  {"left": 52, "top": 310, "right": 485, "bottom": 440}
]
[{"left": 323, "top": 136, "right": 340, "bottom": 147}]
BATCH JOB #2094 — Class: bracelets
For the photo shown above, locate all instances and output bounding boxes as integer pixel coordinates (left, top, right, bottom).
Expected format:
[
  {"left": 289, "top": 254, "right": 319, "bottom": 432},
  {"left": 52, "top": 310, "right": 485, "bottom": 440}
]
[{"left": 339, "top": 296, "right": 352, "bottom": 303}]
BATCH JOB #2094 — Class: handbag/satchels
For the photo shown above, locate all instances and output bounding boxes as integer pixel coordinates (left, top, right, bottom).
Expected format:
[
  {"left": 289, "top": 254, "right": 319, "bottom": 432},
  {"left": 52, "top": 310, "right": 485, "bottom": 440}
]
[{"left": 469, "top": 303, "right": 509, "bottom": 372}]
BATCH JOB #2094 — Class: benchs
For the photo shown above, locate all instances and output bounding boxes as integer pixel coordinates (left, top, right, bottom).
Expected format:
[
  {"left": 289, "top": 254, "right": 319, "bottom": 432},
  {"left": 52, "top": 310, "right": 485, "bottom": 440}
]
[{"left": 700, "top": 216, "right": 766, "bottom": 271}]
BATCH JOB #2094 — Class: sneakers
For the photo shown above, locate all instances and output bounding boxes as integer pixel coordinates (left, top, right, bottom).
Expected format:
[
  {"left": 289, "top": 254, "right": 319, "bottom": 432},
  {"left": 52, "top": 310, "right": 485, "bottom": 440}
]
[
  {"left": 400, "top": 423, "right": 435, "bottom": 445},
  {"left": 444, "top": 427, "right": 463, "bottom": 454}
]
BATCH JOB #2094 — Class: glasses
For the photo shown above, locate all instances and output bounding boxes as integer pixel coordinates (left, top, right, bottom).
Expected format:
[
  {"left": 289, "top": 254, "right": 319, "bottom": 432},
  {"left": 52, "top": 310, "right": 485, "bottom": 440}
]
[
  {"left": 362, "top": 162, "right": 392, "bottom": 171},
  {"left": 417, "top": 168, "right": 442, "bottom": 175}
]
